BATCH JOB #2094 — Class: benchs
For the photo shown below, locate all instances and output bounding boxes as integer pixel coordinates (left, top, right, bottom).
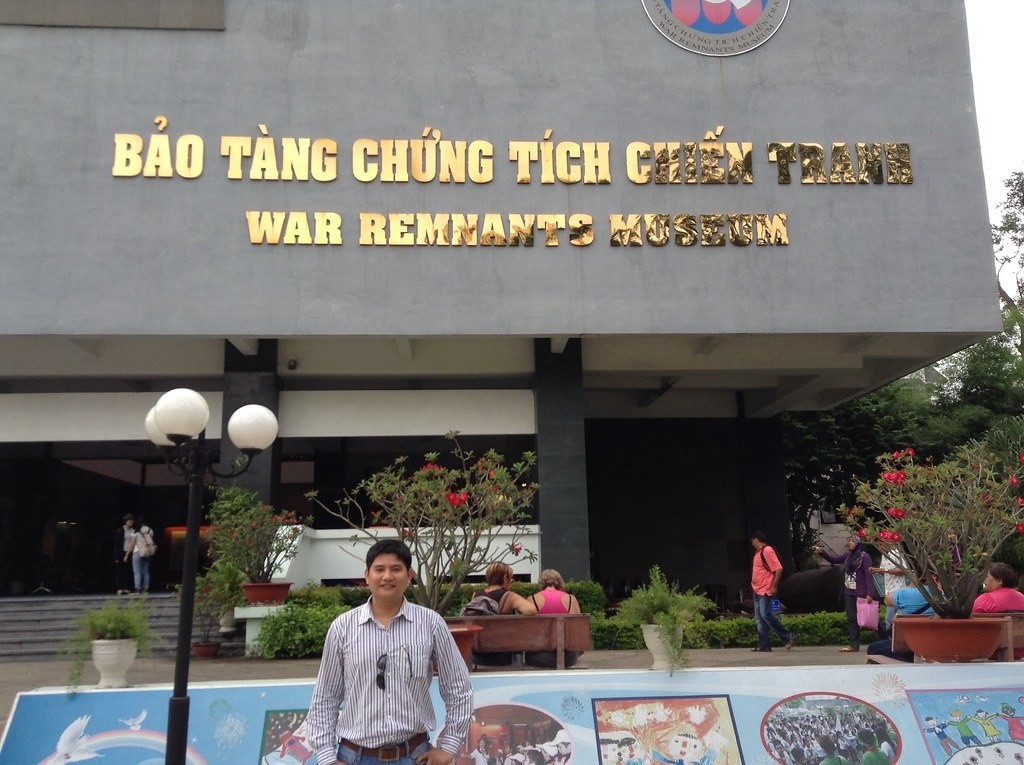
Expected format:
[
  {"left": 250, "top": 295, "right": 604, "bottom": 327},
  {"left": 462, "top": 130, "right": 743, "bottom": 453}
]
[
  {"left": 442, "top": 612, "right": 592, "bottom": 672},
  {"left": 863, "top": 610, "right": 1024, "bottom": 665}
]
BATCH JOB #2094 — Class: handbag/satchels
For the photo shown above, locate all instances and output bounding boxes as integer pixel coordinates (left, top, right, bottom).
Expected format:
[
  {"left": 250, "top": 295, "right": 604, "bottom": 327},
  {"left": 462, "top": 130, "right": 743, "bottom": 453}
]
[
  {"left": 856, "top": 597, "right": 879, "bottom": 631},
  {"left": 887, "top": 624, "right": 892, "bottom": 638}
]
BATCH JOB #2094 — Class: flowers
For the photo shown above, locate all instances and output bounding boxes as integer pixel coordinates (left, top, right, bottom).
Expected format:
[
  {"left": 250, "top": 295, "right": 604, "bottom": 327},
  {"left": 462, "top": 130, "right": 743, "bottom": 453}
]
[
  {"left": 175, "top": 579, "right": 228, "bottom": 643},
  {"left": 224, "top": 504, "right": 313, "bottom": 582},
  {"left": 838, "top": 434, "right": 1024, "bottom": 616},
  {"left": 295, "top": 427, "right": 541, "bottom": 620}
]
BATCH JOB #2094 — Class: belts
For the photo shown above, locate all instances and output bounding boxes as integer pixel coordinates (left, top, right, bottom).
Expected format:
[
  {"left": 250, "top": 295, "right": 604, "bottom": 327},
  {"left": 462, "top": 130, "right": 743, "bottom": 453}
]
[{"left": 341, "top": 732, "right": 430, "bottom": 761}]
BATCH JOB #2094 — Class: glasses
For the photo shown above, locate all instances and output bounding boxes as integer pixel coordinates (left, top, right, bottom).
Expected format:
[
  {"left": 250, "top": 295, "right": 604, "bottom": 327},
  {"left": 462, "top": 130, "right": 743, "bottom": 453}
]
[{"left": 376, "top": 654, "right": 387, "bottom": 692}]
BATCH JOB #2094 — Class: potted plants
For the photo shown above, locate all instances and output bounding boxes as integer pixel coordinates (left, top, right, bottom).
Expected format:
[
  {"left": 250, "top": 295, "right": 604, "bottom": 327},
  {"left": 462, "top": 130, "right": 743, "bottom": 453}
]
[
  {"left": 616, "top": 564, "right": 719, "bottom": 675},
  {"left": 56, "top": 588, "right": 163, "bottom": 696}
]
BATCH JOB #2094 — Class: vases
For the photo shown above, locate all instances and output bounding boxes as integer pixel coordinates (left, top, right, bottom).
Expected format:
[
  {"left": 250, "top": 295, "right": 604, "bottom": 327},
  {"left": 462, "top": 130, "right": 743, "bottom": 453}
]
[
  {"left": 893, "top": 617, "right": 1008, "bottom": 667},
  {"left": 238, "top": 581, "right": 292, "bottom": 608},
  {"left": 428, "top": 624, "right": 483, "bottom": 675},
  {"left": 191, "top": 642, "right": 221, "bottom": 660}
]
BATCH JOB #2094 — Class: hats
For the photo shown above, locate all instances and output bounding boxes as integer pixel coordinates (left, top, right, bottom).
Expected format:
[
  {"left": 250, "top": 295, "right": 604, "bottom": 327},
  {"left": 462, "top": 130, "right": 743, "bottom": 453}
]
[
  {"left": 515, "top": 745, "right": 533, "bottom": 751},
  {"left": 498, "top": 748, "right": 503, "bottom": 755},
  {"left": 536, "top": 741, "right": 559, "bottom": 756},
  {"left": 123, "top": 514, "right": 135, "bottom": 520},
  {"left": 550, "top": 729, "right": 571, "bottom": 747},
  {"left": 508, "top": 753, "right": 525, "bottom": 762}
]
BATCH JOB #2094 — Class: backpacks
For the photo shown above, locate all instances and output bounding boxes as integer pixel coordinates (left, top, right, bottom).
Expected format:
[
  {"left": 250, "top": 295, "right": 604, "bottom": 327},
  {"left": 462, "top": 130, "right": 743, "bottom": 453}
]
[
  {"left": 760, "top": 544, "right": 786, "bottom": 574},
  {"left": 139, "top": 527, "right": 157, "bottom": 557},
  {"left": 458, "top": 587, "right": 507, "bottom": 616}
]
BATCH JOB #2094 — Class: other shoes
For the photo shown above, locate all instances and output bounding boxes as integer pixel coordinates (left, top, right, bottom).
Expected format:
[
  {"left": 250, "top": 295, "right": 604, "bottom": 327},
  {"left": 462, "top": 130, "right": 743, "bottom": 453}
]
[
  {"left": 839, "top": 646, "right": 859, "bottom": 652},
  {"left": 751, "top": 647, "right": 773, "bottom": 652},
  {"left": 117, "top": 589, "right": 130, "bottom": 595},
  {"left": 785, "top": 633, "right": 793, "bottom": 648},
  {"left": 134, "top": 591, "right": 148, "bottom": 595}
]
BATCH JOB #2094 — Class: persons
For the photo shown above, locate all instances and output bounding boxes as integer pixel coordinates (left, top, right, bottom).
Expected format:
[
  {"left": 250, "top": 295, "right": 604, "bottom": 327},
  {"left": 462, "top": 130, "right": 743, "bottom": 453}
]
[
  {"left": 307, "top": 539, "right": 474, "bottom": 765},
  {"left": 472, "top": 562, "right": 584, "bottom": 669},
  {"left": 750, "top": 531, "right": 793, "bottom": 652},
  {"left": 811, "top": 534, "right": 888, "bottom": 652},
  {"left": 115, "top": 514, "right": 153, "bottom": 595},
  {"left": 867, "top": 530, "right": 1024, "bottom": 663}
]
